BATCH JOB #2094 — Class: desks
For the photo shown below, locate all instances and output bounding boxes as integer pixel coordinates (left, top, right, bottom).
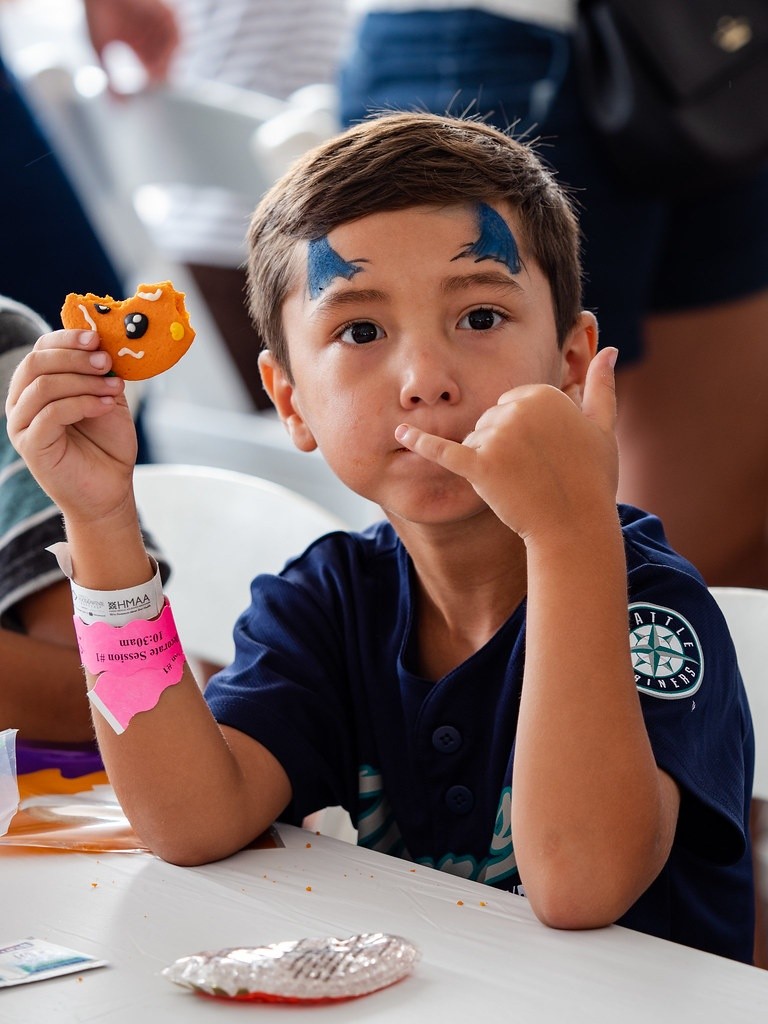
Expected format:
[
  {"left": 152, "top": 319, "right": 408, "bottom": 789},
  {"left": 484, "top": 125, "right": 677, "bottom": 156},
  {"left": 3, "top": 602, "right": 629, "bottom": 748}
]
[{"left": 0, "top": 780, "right": 768, "bottom": 1024}]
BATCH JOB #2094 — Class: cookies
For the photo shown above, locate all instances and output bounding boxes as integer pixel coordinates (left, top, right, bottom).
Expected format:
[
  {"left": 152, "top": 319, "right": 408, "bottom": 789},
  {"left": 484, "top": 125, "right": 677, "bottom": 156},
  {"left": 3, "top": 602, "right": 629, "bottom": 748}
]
[{"left": 60, "top": 280, "right": 197, "bottom": 381}]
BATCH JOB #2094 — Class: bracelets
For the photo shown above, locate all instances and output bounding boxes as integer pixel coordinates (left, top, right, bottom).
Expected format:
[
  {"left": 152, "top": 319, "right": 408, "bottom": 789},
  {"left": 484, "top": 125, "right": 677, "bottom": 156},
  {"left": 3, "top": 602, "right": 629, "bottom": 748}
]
[
  {"left": 73, "top": 598, "right": 188, "bottom": 736},
  {"left": 45, "top": 540, "right": 164, "bottom": 627}
]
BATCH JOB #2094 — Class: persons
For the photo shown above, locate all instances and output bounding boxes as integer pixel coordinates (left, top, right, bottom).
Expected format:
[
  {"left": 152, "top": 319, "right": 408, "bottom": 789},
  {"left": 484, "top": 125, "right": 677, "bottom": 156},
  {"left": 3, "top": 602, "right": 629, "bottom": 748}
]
[{"left": 1, "top": 1, "right": 768, "bottom": 965}]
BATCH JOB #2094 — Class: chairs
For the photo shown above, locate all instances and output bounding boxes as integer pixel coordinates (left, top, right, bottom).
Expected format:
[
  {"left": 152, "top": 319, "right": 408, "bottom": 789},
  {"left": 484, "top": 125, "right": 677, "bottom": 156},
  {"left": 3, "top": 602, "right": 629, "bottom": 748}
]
[{"left": 129, "top": 463, "right": 768, "bottom": 801}]
